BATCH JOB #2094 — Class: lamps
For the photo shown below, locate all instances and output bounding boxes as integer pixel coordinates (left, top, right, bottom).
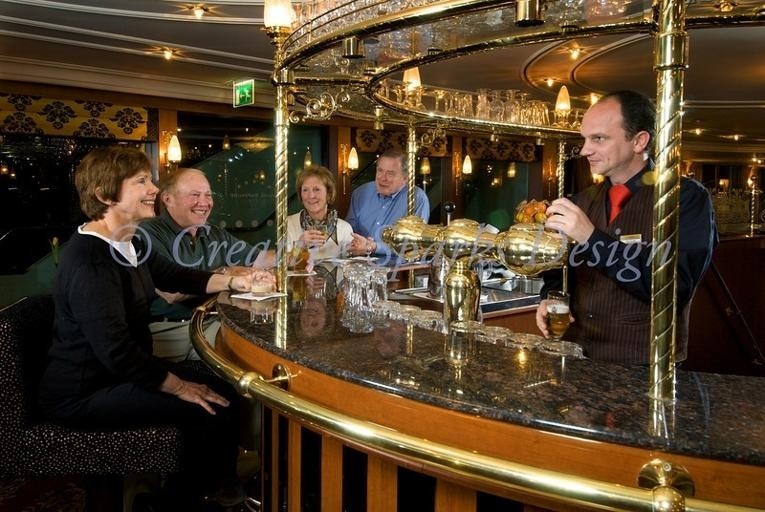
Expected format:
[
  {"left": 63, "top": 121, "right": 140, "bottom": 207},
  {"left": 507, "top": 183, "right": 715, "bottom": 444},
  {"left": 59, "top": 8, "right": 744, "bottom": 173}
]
[
  {"left": 342, "top": 145, "right": 359, "bottom": 197},
  {"left": 264, "top": 1, "right": 295, "bottom": 353},
  {"left": 166, "top": 134, "right": 184, "bottom": 171},
  {"left": 404, "top": 67, "right": 423, "bottom": 357},
  {"left": 455, "top": 152, "right": 472, "bottom": 196},
  {"left": 552, "top": 85, "right": 569, "bottom": 292},
  {"left": 420, "top": 157, "right": 431, "bottom": 193}
]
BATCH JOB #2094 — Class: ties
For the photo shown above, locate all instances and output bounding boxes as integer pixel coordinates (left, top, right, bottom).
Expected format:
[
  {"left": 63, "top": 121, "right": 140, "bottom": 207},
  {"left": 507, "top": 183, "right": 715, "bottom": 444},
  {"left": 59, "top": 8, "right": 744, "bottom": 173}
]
[{"left": 607, "top": 184, "right": 632, "bottom": 225}]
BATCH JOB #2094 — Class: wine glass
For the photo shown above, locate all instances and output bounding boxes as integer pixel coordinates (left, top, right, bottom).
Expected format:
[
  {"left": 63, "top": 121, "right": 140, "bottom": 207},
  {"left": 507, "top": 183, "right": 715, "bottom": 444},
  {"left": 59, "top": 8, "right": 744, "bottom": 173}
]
[
  {"left": 374, "top": 267, "right": 390, "bottom": 299},
  {"left": 342, "top": 266, "right": 373, "bottom": 334}
]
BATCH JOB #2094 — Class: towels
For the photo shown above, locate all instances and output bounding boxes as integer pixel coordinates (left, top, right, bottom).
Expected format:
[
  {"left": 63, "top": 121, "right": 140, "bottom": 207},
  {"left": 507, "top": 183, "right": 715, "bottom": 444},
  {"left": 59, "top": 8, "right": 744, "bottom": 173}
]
[
  {"left": 287, "top": 269, "right": 317, "bottom": 276},
  {"left": 231, "top": 292, "right": 288, "bottom": 301},
  {"left": 349, "top": 256, "right": 379, "bottom": 261}
]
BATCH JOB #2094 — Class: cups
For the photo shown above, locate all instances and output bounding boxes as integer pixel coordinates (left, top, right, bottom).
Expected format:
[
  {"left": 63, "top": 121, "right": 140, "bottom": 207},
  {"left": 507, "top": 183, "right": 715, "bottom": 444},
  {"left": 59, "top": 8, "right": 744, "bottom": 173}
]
[
  {"left": 506, "top": 333, "right": 543, "bottom": 351},
  {"left": 292, "top": 276, "right": 309, "bottom": 308},
  {"left": 450, "top": 320, "right": 483, "bottom": 337},
  {"left": 398, "top": 303, "right": 422, "bottom": 321},
  {"left": 313, "top": 224, "right": 327, "bottom": 244},
  {"left": 251, "top": 301, "right": 273, "bottom": 324},
  {"left": 416, "top": 310, "right": 442, "bottom": 334},
  {"left": 542, "top": 341, "right": 582, "bottom": 361},
  {"left": 445, "top": 321, "right": 468, "bottom": 367},
  {"left": 251, "top": 269, "right": 267, "bottom": 297},
  {"left": 546, "top": 290, "right": 570, "bottom": 342},
  {"left": 476, "top": 326, "right": 510, "bottom": 343},
  {"left": 293, "top": 241, "right": 309, "bottom": 274},
  {"left": 370, "top": 300, "right": 400, "bottom": 329}
]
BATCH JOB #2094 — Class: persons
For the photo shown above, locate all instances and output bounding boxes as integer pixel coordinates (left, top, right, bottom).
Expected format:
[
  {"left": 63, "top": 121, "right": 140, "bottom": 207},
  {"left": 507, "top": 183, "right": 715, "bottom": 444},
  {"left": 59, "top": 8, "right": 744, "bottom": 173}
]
[
  {"left": 35, "top": 144, "right": 277, "bottom": 512},
  {"left": 343, "top": 148, "right": 431, "bottom": 279},
  {"left": 534, "top": 88, "right": 719, "bottom": 371},
  {"left": 287, "top": 164, "right": 354, "bottom": 265},
  {"left": 135, "top": 167, "right": 277, "bottom": 361}
]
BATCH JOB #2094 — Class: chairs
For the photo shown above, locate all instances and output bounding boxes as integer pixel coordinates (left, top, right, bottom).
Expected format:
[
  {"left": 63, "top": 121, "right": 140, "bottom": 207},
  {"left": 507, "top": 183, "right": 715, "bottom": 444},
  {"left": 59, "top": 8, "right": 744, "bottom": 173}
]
[{"left": 0, "top": 294, "right": 180, "bottom": 512}]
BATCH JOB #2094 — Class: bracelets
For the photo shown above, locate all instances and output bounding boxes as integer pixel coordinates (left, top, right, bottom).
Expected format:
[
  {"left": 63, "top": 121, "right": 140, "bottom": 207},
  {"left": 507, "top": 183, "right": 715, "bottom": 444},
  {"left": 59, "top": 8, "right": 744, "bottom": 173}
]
[
  {"left": 228, "top": 276, "right": 233, "bottom": 291},
  {"left": 171, "top": 380, "right": 188, "bottom": 395}
]
